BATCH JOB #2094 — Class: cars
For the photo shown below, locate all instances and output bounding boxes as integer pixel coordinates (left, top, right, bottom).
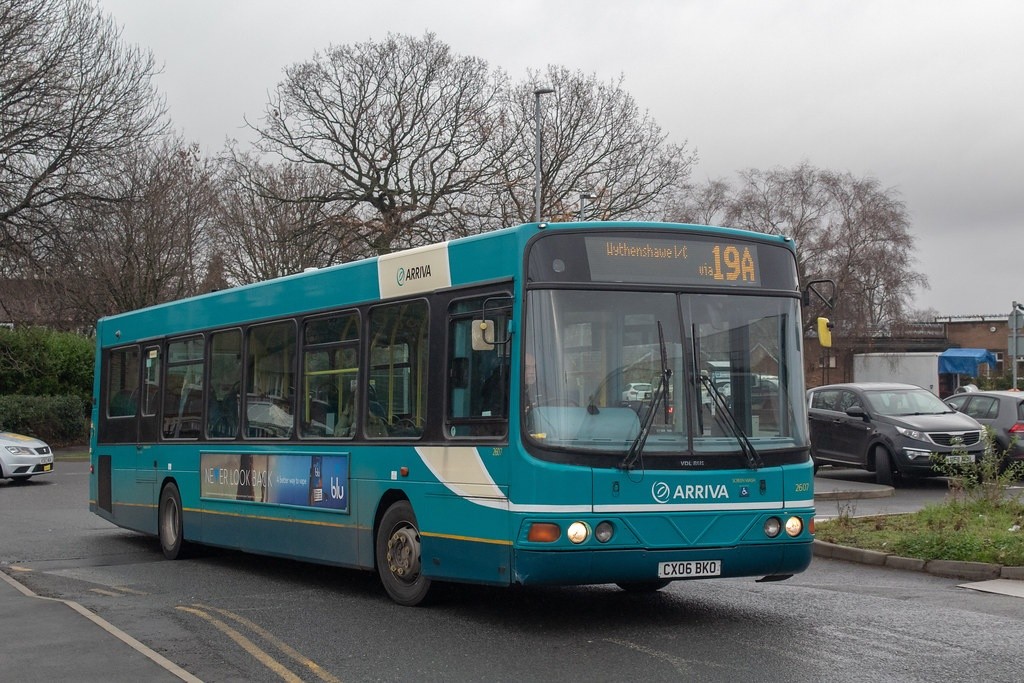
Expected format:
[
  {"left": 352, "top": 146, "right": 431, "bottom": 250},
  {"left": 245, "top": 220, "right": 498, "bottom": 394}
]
[
  {"left": 942, "top": 390, "right": 1024, "bottom": 476},
  {"left": 655, "top": 362, "right": 783, "bottom": 429},
  {"left": 621, "top": 382, "right": 655, "bottom": 403},
  {"left": 0, "top": 431, "right": 54, "bottom": 480}
]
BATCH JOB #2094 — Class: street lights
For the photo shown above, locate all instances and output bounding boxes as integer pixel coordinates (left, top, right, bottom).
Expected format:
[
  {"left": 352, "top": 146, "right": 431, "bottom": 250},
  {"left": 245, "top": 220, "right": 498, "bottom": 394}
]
[
  {"left": 533, "top": 87, "right": 555, "bottom": 223},
  {"left": 1012, "top": 300, "right": 1024, "bottom": 392},
  {"left": 580, "top": 192, "right": 594, "bottom": 220}
]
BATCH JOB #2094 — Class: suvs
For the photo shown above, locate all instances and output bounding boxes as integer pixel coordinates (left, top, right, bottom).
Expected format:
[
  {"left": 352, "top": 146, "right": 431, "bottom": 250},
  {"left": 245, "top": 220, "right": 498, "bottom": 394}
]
[{"left": 807, "top": 383, "right": 989, "bottom": 487}]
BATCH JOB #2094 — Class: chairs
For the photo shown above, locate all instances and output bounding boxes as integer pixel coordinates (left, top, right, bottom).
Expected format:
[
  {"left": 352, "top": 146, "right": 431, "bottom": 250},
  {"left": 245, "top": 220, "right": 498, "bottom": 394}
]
[
  {"left": 977, "top": 402, "right": 988, "bottom": 416},
  {"left": 113, "top": 381, "right": 425, "bottom": 436}
]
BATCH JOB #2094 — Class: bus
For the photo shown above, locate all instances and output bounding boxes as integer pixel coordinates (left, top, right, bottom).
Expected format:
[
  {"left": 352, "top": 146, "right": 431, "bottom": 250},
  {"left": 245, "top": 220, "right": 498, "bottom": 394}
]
[{"left": 84, "top": 220, "right": 837, "bottom": 601}]
